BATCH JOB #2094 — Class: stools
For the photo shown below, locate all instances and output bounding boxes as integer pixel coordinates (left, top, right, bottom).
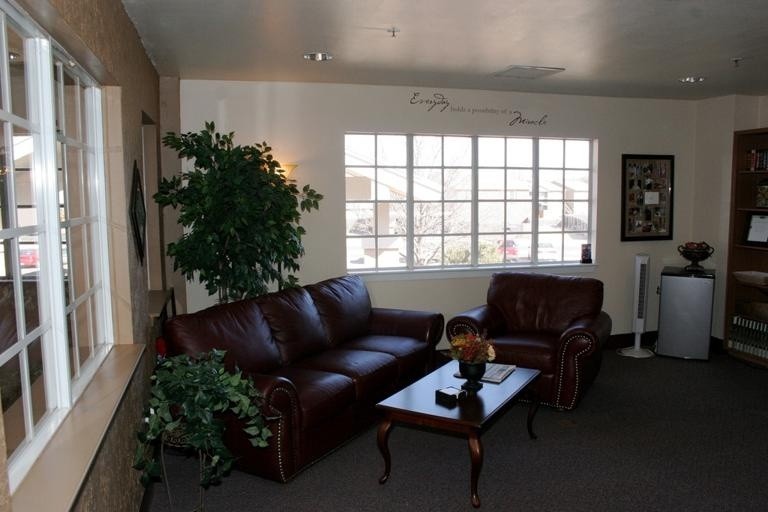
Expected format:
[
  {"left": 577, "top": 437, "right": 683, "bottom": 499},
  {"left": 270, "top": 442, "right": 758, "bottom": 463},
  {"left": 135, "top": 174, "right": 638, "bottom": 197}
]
[{"left": 161, "top": 431, "right": 210, "bottom": 511}]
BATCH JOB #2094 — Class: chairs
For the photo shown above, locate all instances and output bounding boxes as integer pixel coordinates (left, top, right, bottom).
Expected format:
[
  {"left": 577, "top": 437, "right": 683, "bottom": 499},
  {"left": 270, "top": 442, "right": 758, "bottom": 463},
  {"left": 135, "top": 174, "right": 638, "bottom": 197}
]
[{"left": 445, "top": 273, "right": 613, "bottom": 411}]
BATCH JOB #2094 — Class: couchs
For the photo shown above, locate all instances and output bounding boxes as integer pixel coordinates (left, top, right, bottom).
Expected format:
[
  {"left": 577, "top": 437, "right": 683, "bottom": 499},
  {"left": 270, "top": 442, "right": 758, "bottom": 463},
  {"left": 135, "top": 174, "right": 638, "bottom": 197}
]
[{"left": 157, "top": 273, "right": 443, "bottom": 484}]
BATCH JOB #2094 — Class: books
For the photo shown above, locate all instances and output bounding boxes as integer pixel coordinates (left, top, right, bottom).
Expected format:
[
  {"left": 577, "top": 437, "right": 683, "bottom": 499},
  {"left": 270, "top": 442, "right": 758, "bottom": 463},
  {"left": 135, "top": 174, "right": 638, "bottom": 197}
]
[
  {"left": 744, "top": 147, "right": 768, "bottom": 172},
  {"left": 453, "top": 361, "right": 515, "bottom": 383},
  {"left": 727, "top": 314, "right": 768, "bottom": 360}
]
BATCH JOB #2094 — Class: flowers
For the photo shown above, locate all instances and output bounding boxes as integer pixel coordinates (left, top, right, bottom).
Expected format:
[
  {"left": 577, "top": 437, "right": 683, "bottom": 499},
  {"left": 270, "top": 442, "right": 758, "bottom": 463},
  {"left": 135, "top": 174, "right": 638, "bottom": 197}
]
[{"left": 450, "top": 332, "right": 497, "bottom": 363}]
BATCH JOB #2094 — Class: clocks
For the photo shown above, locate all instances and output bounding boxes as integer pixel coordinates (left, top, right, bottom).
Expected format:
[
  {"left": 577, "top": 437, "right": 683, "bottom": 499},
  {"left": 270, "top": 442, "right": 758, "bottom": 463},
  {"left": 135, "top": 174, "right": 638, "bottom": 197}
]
[{"left": 130, "top": 160, "right": 146, "bottom": 267}]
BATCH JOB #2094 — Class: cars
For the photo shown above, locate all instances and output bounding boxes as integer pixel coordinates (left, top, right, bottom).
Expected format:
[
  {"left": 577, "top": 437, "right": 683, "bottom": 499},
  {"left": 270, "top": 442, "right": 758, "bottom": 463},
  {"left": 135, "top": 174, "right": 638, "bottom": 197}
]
[
  {"left": 10, "top": 232, "right": 69, "bottom": 279},
  {"left": 526, "top": 242, "right": 559, "bottom": 262},
  {"left": 347, "top": 216, "right": 374, "bottom": 236},
  {"left": 496, "top": 240, "right": 519, "bottom": 256}
]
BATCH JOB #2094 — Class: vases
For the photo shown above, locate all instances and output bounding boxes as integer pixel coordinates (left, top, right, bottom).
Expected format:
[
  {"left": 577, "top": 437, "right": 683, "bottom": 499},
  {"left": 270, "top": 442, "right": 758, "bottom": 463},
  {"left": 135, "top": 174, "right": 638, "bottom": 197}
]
[{"left": 457, "top": 361, "right": 484, "bottom": 390}]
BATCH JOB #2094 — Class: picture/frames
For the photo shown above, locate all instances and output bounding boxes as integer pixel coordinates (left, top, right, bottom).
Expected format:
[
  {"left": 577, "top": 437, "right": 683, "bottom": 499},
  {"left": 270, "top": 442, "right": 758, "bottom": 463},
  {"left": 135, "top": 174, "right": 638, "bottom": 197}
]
[{"left": 620, "top": 154, "right": 676, "bottom": 242}]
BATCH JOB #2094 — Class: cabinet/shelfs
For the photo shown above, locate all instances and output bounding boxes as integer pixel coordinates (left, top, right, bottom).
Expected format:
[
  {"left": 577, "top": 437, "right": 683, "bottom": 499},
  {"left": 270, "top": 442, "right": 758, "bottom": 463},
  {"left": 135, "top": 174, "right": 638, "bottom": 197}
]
[
  {"left": 655, "top": 266, "right": 716, "bottom": 361},
  {"left": 723, "top": 124, "right": 768, "bottom": 368}
]
